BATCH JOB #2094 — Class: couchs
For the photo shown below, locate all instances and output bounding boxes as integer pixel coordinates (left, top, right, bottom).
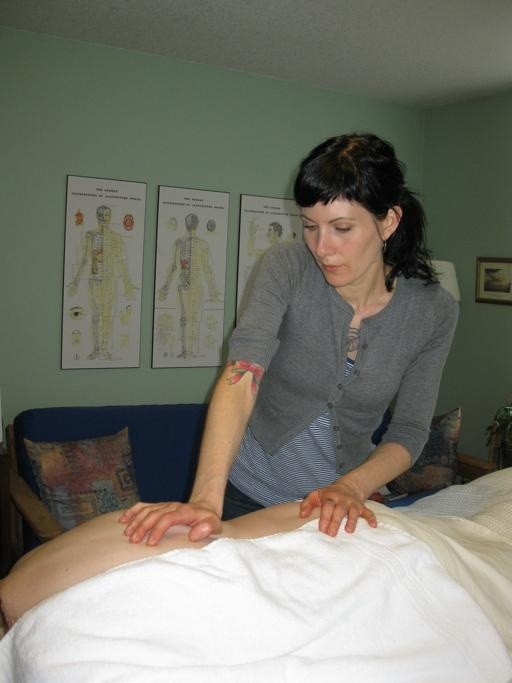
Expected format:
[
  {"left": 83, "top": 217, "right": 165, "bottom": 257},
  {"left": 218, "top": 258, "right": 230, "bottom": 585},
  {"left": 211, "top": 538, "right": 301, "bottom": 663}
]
[{"left": 2, "top": 405, "right": 498, "bottom": 554}]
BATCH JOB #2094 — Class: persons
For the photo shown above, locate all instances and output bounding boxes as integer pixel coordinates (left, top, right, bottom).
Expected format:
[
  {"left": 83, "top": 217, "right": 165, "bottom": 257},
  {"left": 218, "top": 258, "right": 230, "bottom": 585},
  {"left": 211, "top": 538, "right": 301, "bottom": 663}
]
[
  {"left": 1, "top": 496, "right": 331, "bottom": 640},
  {"left": 117, "top": 128, "right": 463, "bottom": 548},
  {"left": 65, "top": 203, "right": 142, "bottom": 361},
  {"left": 154, "top": 210, "right": 222, "bottom": 361},
  {"left": 248, "top": 216, "right": 285, "bottom": 258}
]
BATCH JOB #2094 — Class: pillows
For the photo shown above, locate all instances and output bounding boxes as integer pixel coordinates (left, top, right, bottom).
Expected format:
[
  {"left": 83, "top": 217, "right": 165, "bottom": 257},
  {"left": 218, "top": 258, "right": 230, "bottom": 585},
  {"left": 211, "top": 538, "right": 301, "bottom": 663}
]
[
  {"left": 24, "top": 428, "right": 141, "bottom": 534},
  {"left": 388, "top": 407, "right": 463, "bottom": 494}
]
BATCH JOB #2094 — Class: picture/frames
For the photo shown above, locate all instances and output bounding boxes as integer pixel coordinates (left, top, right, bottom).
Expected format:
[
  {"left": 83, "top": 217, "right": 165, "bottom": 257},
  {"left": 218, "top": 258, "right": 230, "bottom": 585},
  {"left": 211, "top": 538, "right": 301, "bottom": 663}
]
[{"left": 474, "top": 257, "right": 512, "bottom": 306}]
